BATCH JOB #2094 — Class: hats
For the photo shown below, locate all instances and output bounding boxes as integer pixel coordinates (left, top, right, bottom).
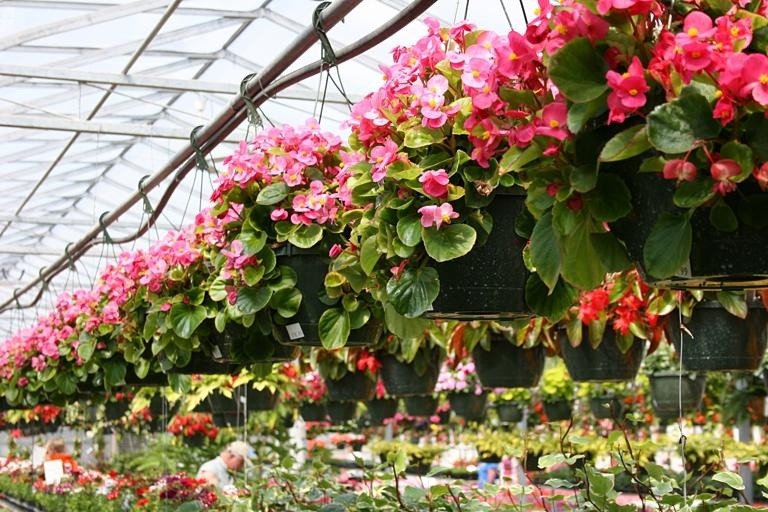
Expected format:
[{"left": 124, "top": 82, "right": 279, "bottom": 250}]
[{"left": 227, "top": 441, "right": 253, "bottom": 468}]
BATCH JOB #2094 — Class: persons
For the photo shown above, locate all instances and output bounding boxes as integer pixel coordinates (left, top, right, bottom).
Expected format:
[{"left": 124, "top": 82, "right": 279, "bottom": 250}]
[{"left": 195, "top": 441, "right": 258, "bottom": 502}]
